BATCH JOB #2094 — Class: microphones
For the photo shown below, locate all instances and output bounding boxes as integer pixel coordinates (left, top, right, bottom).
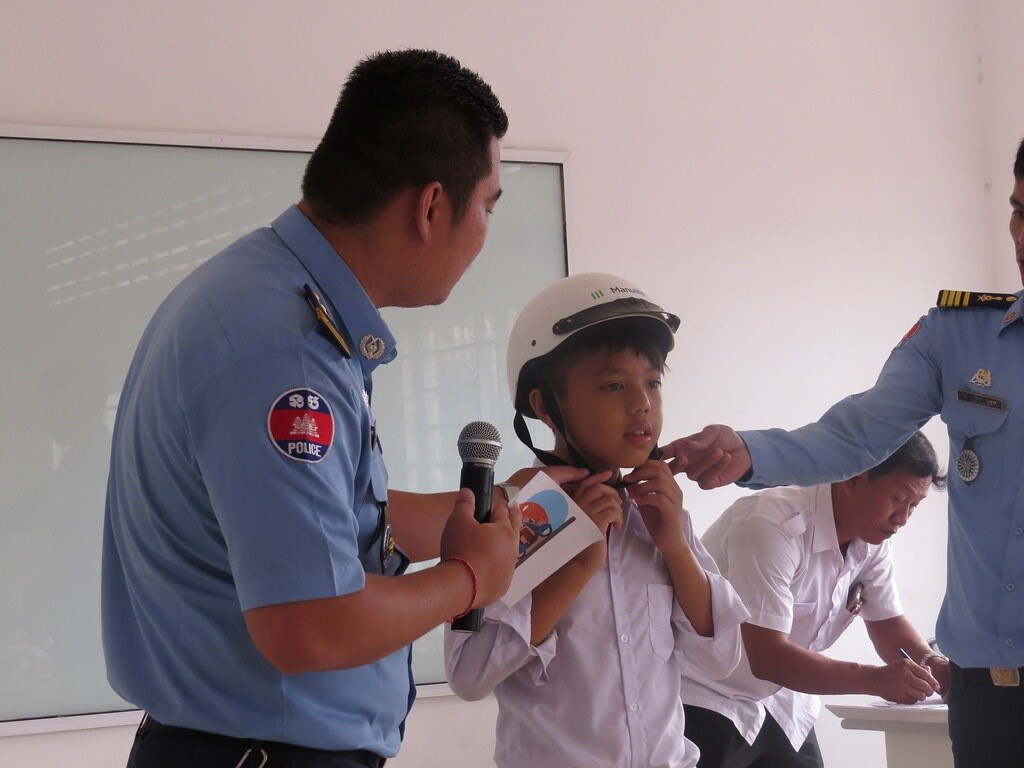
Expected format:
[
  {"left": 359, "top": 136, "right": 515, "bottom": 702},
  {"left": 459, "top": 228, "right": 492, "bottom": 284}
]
[{"left": 448, "top": 422, "right": 502, "bottom": 635}]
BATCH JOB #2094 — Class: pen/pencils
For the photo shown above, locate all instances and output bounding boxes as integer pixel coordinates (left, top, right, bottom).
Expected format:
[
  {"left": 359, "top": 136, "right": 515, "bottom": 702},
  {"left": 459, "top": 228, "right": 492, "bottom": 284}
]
[{"left": 898, "top": 647, "right": 945, "bottom": 699}]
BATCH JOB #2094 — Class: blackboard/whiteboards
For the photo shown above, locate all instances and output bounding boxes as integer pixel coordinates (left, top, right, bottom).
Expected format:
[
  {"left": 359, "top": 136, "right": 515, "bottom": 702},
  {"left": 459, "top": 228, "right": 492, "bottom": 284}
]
[{"left": 3, "top": 121, "right": 571, "bottom": 737}]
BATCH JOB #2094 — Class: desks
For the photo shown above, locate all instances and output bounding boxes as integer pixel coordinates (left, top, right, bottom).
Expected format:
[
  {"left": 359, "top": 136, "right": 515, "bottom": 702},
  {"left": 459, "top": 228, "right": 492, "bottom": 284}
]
[{"left": 826, "top": 699, "right": 955, "bottom": 768}]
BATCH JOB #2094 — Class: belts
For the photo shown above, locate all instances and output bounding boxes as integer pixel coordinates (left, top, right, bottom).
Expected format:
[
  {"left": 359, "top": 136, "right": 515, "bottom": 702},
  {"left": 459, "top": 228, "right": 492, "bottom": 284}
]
[{"left": 140, "top": 714, "right": 384, "bottom": 768}]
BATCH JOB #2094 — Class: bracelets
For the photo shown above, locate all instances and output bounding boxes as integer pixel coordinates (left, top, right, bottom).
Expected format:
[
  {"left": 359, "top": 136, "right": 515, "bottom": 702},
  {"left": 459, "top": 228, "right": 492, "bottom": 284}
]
[
  {"left": 437, "top": 555, "right": 479, "bottom": 623},
  {"left": 916, "top": 653, "right": 941, "bottom": 671}
]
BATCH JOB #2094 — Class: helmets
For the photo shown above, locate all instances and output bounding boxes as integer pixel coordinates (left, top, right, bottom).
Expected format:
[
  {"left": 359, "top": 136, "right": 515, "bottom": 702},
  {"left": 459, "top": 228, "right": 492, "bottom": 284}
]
[{"left": 506, "top": 269, "right": 682, "bottom": 418}]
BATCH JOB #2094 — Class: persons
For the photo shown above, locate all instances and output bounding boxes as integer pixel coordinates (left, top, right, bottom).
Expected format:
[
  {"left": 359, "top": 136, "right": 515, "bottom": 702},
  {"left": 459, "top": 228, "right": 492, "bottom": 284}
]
[
  {"left": 443, "top": 274, "right": 752, "bottom": 768},
  {"left": 100, "top": 46, "right": 588, "bottom": 768},
  {"left": 657, "top": 133, "right": 1024, "bottom": 768},
  {"left": 676, "top": 430, "right": 951, "bottom": 768}
]
